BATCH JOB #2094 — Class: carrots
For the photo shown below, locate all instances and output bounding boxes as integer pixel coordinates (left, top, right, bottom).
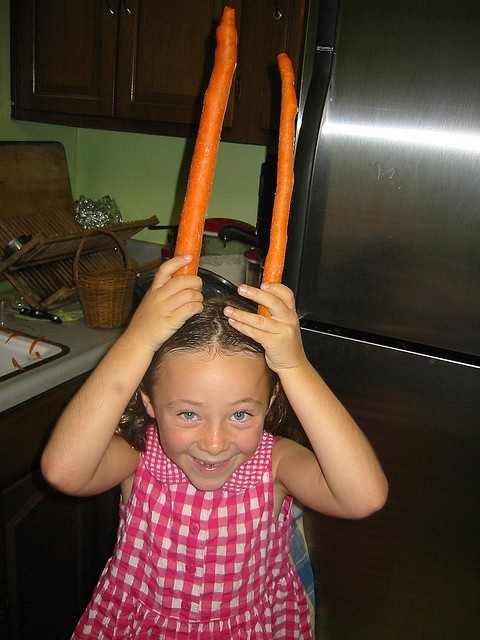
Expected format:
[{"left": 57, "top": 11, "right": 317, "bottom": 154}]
[
  {"left": 172, "top": 7, "right": 241, "bottom": 280},
  {"left": 257, "top": 52, "right": 300, "bottom": 322}
]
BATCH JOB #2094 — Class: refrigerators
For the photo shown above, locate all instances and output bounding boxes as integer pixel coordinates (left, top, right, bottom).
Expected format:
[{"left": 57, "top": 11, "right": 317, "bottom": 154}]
[{"left": 268, "top": 1, "right": 479, "bottom": 640}]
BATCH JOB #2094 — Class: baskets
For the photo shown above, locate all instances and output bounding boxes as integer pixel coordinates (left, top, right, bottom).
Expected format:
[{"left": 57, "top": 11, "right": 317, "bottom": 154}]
[{"left": 74, "top": 228, "right": 134, "bottom": 329}]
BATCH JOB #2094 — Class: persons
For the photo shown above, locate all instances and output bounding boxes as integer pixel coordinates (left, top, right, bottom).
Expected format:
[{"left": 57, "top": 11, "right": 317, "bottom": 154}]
[{"left": 40, "top": 255, "right": 389, "bottom": 634}]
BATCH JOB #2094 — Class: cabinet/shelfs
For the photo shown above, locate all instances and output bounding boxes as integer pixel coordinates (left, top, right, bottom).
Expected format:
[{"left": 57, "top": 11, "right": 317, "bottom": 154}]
[
  {"left": 0, "top": 372, "right": 121, "bottom": 639},
  {"left": 247, "top": 0, "right": 305, "bottom": 148},
  {"left": 10, "top": 1, "right": 255, "bottom": 145}
]
